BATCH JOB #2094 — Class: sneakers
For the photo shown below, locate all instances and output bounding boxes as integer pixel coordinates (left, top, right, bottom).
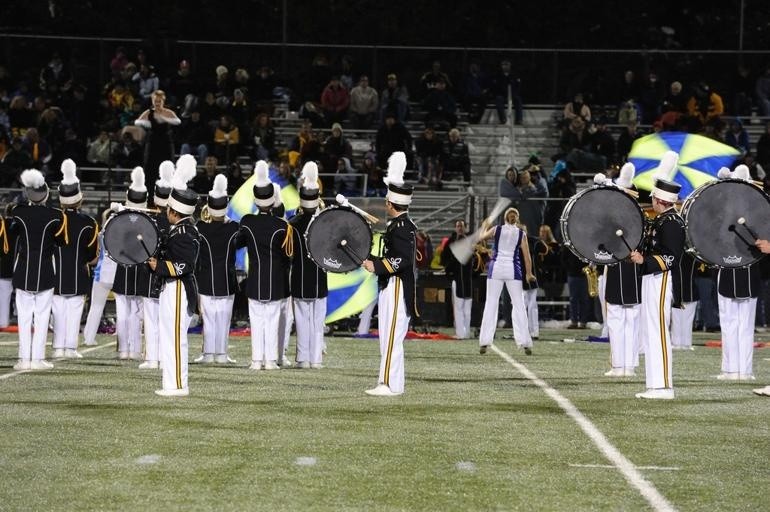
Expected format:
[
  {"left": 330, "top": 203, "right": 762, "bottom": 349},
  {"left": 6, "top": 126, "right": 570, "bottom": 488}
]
[
  {"left": 523, "top": 347, "right": 532, "bottom": 355},
  {"left": 194, "top": 354, "right": 213, "bottom": 363},
  {"left": 671, "top": 344, "right": 694, "bottom": 351},
  {"left": 568, "top": 323, "right": 587, "bottom": 329},
  {"left": 116, "top": 350, "right": 160, "bottom": 369},
  {"left": 636, "top": 388, "right": 675, "bottom": 400},
  {"left": 365, "top": 384, "right": 401, "bottom": 396},
  {"left": 215, "top": 354, "right": 236, "bottom": 363},
  {"left": 604, "top": 366, "right": 637, "bottom": 377},
  {"left": 249, "top": 350, "right": 328, "bottom": 371},
  {"left": 154, "top": 387, "right": 189, "bottom": 397},
  {"left": 11, "top": 341, "right": 97, "bottom": 370},
  {"left": 480, "top": 344, "right": 487, "bottom": 355},
  {"left": 752, "top": 385, "right": 770, "bottom": 396},
  {"left": 718, "top": 372, "right": 756, "bottom": 381}
]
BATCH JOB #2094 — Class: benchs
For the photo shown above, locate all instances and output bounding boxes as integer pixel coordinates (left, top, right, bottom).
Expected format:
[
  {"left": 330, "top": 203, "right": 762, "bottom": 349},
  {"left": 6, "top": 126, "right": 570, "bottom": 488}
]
[{"left": 1, "top": 66, "right": 769, "bottom": 245}]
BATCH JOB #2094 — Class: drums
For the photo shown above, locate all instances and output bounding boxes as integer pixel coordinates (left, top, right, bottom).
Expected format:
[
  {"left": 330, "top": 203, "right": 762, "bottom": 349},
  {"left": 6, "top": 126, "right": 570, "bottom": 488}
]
[
  {"left": 101, "top": 208, "right": 162, "bottom": 267},
  {"left": 304, "top": 205, "right": 373, "bottom": 273},
  {"left": 561, "top": 186, "right": 645, "bottom": 266},
  {"left": 680, "top": 179, "right": 770, "bottom": 270}
]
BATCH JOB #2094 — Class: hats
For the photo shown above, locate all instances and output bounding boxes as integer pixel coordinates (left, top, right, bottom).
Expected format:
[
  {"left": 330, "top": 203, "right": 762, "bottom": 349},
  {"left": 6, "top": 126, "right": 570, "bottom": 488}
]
[
  {"left": 649, "top": 179, "right": 682, "bottom": 204},
  {"left": 127, "top": 189, "right": 148, "bottom": 203},
  {"left": 168, "top": 189, "right": 200, "bottom": 215},
  {"left": 300, "top": 186, "right": 319, "bottom": 208},
  {"left": 386, "top": 183, "right": 414, "bottom": 206},
  {"left": 271, "top": 203, "right": 285, "bottom": 217},
  {"left": 58, "top": 182, "right": 82, "bottom": 205},
  {"left": 27, "top": 181, "right": 49, "bottom": 205},
  {"left": 154, "top": 184, "right": 171, "bottom": 208},
  {"left": 208, "top": 194, "right": 229, "bottom": 217},
  {"left": 253, "top": 184, "right": 274, "bottom": 207}
]
